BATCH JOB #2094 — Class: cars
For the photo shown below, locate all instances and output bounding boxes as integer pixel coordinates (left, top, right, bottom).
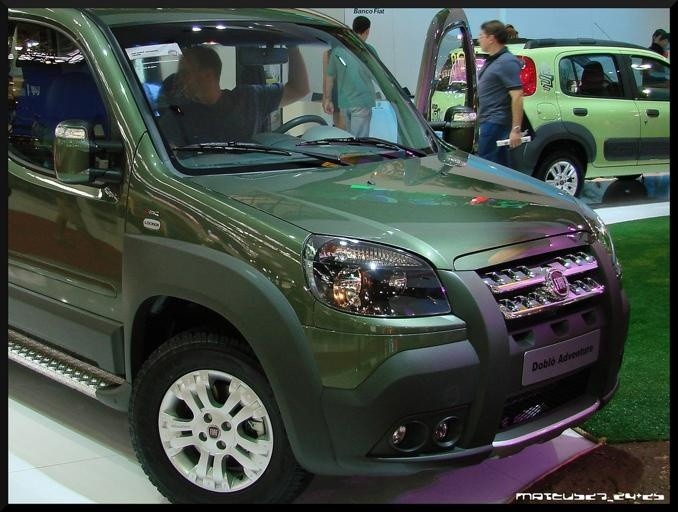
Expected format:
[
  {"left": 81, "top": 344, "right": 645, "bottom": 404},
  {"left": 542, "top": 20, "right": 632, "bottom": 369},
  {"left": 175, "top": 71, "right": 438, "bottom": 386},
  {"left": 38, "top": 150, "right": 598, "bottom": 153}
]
[
  {"left": 5, "top": 8, "right": 632, "bottom": 505},
  {"left": 426, "top": 37, "right": 669, "bottom": 198}
]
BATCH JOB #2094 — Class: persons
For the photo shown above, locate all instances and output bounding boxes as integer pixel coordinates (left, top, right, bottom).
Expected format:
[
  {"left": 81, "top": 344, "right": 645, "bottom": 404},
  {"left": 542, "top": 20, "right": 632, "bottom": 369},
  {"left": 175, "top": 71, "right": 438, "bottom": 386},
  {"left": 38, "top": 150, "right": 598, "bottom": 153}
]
[
  {"left": 324, "top": 15, "right": 379, "bottom": 138},
  {"left": 475, "top": 19, "right": 525, "bottom": 169},
  {"left": 322, "top": 46, "right": 347, "bottom": 131},
  {"left": 157, "top": 46, "right": 309, "bottom": 164},
  {"left": 641, "top": 33, "right": 671, "bottom": 85},
  {"left": 638, "top": 28, "right": 667, "bottom": 76}
]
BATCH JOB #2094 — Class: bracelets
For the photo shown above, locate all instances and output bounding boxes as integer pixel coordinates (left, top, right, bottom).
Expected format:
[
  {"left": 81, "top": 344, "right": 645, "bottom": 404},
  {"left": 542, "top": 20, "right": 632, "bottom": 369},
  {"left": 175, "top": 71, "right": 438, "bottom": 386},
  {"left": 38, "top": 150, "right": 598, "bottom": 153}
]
[{"left": 511, "top": 126, "right": 521, "bottom": 131}]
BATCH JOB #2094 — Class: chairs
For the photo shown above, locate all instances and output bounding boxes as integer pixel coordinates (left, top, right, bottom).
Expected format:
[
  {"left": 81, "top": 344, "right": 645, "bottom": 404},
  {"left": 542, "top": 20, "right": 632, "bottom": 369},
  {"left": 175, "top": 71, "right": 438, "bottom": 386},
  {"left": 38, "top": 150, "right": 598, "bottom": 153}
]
[
  {"left": 14, "top": 56, "right": 116, "bottom": 150},
  {"left": 579, "top": 61, "right": 610, "bottom": 96}
]
